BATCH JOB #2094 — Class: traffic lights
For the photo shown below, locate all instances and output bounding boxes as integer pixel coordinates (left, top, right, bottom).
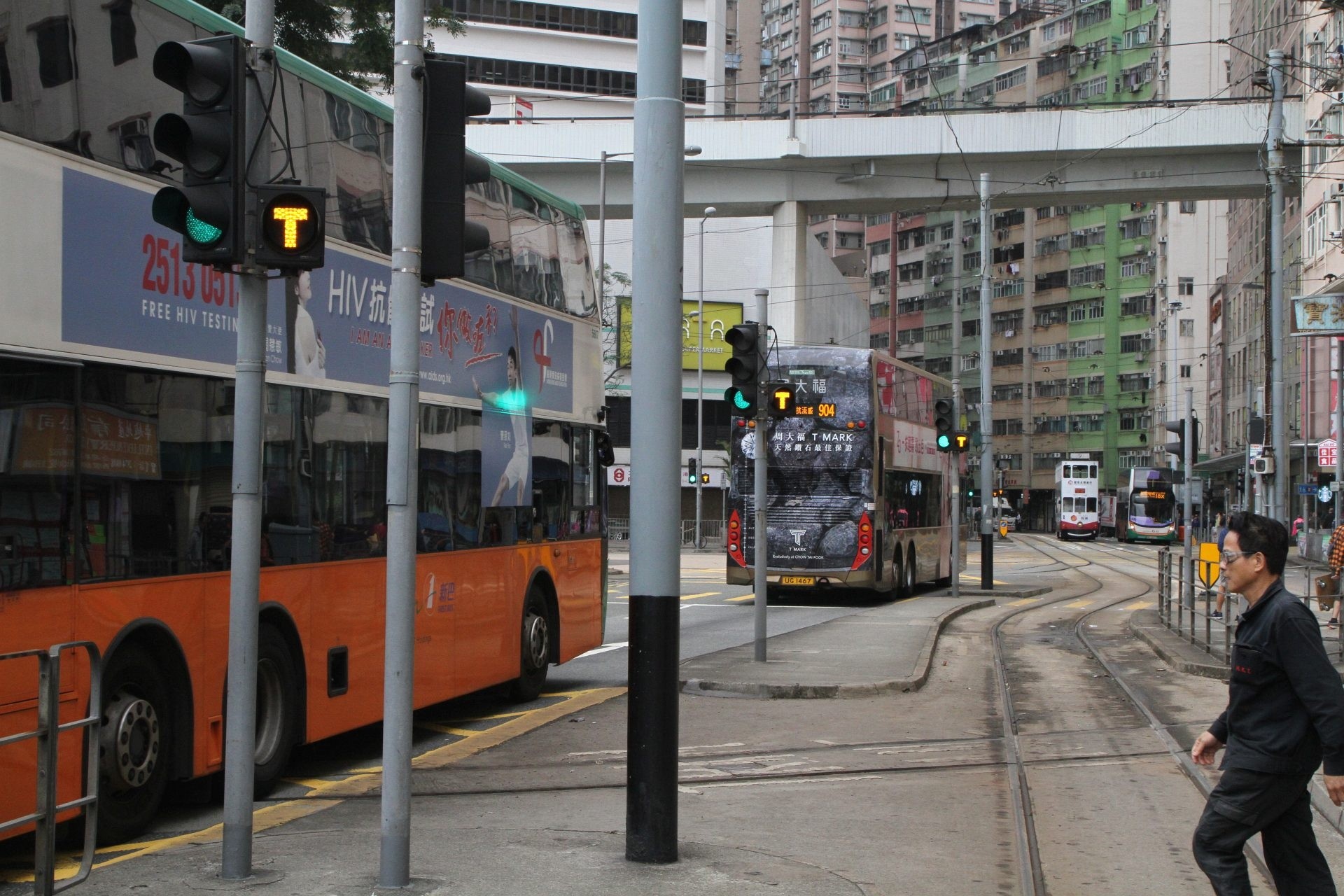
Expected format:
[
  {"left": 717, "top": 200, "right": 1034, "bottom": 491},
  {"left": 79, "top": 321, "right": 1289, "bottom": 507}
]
[
  {"left": 688, "top": 457, "right": 697, "bottom": 484},
  {"left": 702, "top": 473, "right": 710, "bottom": 483},
  {"left": 934, "top": 397, "right": 970, "bottom": 452},
  {"left": 246, "top": 188, "right": 326, "bottom": 271},
  {"left": 724, "top": 323, "right": 758, "bottom": 417},
  {"left": 767, "top": 382, "right": 797, "bottom": 418},
  {"left": 151, "top": 34, "right": 248, "bottom": 266},
  {"left": 416, "top": 59, "right": 493, "bottom": 288},
  {"left": 1160, "top": 417, "right": 1198, "bottom": 465}
]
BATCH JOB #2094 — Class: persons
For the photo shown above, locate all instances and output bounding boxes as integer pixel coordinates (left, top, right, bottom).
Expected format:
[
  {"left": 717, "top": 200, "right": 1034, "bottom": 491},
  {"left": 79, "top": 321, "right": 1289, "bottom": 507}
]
[
  {"left": 423, "top": 508, "right": 581, "bottom": 552},
  {"left": 309, "top": 508, "right": 388, "bottom": 563},
  {"left": 295, "top": 270, "right": 326, "bottom": 380},
  {"left": 974, "top": 506, "right": 981, "bottom": 539},
  {"left": 1292, "top": 508, "right": 1344, "bottom": 542},
  {"left": 1327, "top": 524, "right": 1344, "bottom": 629},
  {"left": 342, "top": 193, "right": 383, "bottom": 253},
  {"left": 1210, "top": 510, "right": 1237, "bottom": 619},
  {"left": 1175, "top": 510, "right": 1227, "bottom": 539},
  {"left": 471, "top": 306, "right": 528, "bottom": 506},
  {"left": 1016, "top": 511, "right": 1022, "bottom": 532},
  {"left": 1192, "top": 513, "right": 1344, "bottom": 896},
  {"left": 156, "top": 513, "right": 281, "bottom": 578}
]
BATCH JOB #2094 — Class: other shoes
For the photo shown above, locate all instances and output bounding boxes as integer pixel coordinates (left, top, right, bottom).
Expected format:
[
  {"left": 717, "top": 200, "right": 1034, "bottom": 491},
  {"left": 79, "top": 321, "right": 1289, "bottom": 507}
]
[
  {"left": 1328, "top": 621, "right": 1339, "bottom": 628},
  {"left": 1235, "top": 615, "right": 1239, "bottom": 622},
  {"left": 1211, "top": 610, "right": 1223, "bottom": 619}
]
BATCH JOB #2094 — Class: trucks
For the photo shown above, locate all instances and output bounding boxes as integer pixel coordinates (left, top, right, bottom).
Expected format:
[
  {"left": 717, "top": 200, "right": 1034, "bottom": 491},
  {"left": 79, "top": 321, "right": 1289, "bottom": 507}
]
[{"left": 975, "top": 497, "right": 1017, "bottom": 531}]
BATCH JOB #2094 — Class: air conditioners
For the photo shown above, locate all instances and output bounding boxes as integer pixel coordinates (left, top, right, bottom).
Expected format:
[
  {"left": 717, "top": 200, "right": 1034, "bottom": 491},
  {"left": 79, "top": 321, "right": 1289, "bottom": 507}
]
[
  {"left": 1321, "top": 100, "right": 1344, "bottom": 144},
  {"left": 1159, "top": 23, "right": 1170, "bottom": 81},
  {"left": 880, "top": 212, "right": 1167, "bottom": 421},
  {"left": 1066, "top": 67, "right": 1077, "bottom": 76},
  {"left": 765, "top": 5, "right": 994, "bottom": 103},
  {"left": 1129, "top": 84, "right": 1138, "bottom": 91}
]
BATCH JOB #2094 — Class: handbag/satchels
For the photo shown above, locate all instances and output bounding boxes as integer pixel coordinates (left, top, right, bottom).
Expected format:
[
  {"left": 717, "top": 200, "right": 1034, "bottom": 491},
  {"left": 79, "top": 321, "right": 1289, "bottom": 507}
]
[{"left": 1326, "top": 543, "right": 1334, "bottom": 556}]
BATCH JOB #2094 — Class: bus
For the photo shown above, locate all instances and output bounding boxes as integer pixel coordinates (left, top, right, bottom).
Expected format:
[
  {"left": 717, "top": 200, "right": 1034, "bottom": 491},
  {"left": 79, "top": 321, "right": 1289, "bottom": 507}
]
[
  {"left": 0, "top": 0, "right": 615, "bottom": 850},
  {"left": 1055, "top": 460, "right": 1101, "bottom": 541},
  {"left": 1115, "top": 465, "right": 1177, "bottom": 545},
  {"left": 723, "top": 347, "right": 970, "bottom": 597}
]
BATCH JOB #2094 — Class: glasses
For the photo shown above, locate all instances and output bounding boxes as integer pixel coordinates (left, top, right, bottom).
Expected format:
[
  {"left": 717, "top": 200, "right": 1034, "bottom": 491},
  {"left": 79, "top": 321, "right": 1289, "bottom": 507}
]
[{"left": 1219, "top": 551, "right": 1257, "bottom": 562}]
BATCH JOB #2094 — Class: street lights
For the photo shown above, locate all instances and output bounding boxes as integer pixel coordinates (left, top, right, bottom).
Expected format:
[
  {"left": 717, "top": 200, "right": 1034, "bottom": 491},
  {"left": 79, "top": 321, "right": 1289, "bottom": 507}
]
[
  {"left": 697, "top": 206, "right": 717, "bottom": 553},
  {"left": 596, "top": 145, "right": 710, "bottom": 336}
]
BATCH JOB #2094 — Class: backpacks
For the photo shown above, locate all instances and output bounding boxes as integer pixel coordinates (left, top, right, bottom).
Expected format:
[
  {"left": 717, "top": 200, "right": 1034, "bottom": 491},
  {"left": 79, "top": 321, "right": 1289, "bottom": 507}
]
[{"left": 1296, "top": 522, "right": 1302, "bottom": 529}]
